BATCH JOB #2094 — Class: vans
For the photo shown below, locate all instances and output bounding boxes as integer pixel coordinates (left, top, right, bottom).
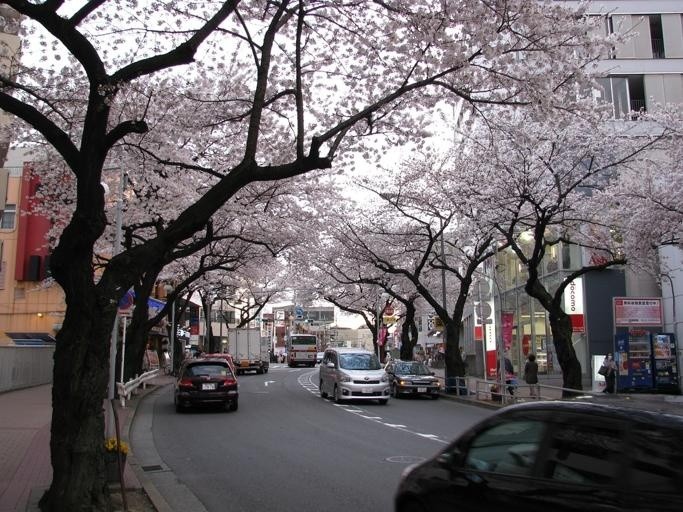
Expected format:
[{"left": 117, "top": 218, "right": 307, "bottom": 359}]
[{"left": 318, "top": 347, "right": 390, "bottom": 405}]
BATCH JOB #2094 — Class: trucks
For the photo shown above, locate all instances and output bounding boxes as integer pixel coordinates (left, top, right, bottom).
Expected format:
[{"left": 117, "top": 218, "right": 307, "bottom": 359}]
[{"left": 227, "top": 327, "right": 269, "bottom": 374}]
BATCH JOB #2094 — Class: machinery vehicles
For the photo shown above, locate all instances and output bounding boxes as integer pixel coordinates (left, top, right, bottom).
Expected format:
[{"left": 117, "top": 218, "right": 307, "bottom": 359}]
[{"left": 286, "top": 321, "right": 317, "bottom": 367}]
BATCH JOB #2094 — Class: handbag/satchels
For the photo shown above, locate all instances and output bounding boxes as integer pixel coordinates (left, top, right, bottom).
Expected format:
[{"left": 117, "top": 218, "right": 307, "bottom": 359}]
[{"left": 597, "top": 365, "right": 607, "bottom": 375}]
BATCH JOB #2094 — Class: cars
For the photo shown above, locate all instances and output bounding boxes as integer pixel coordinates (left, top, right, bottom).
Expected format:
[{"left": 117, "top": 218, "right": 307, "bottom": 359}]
[
  {"left": 173, "top": 353, "right": 238, "bottom": 411},
  {"left": 393, "top": 392, "right": 682, "bottom": 511},
  {"left": 385, "top": 360, "right": 440, "bottom": 399}
]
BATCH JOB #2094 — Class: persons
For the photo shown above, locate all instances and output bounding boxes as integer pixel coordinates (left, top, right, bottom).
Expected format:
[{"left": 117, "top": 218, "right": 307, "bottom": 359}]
[
  {"left": 497, "top": 351, "right": 514, "bottom": 398},
  {"left": 459, "top": 346, "right": 466, "bottom": 375},
  {"left": 524, "top": 355, "right": 538, "bottom": 399},
  {"left": 602, "top": 352, "right": 619, "bottom": 393}
]
